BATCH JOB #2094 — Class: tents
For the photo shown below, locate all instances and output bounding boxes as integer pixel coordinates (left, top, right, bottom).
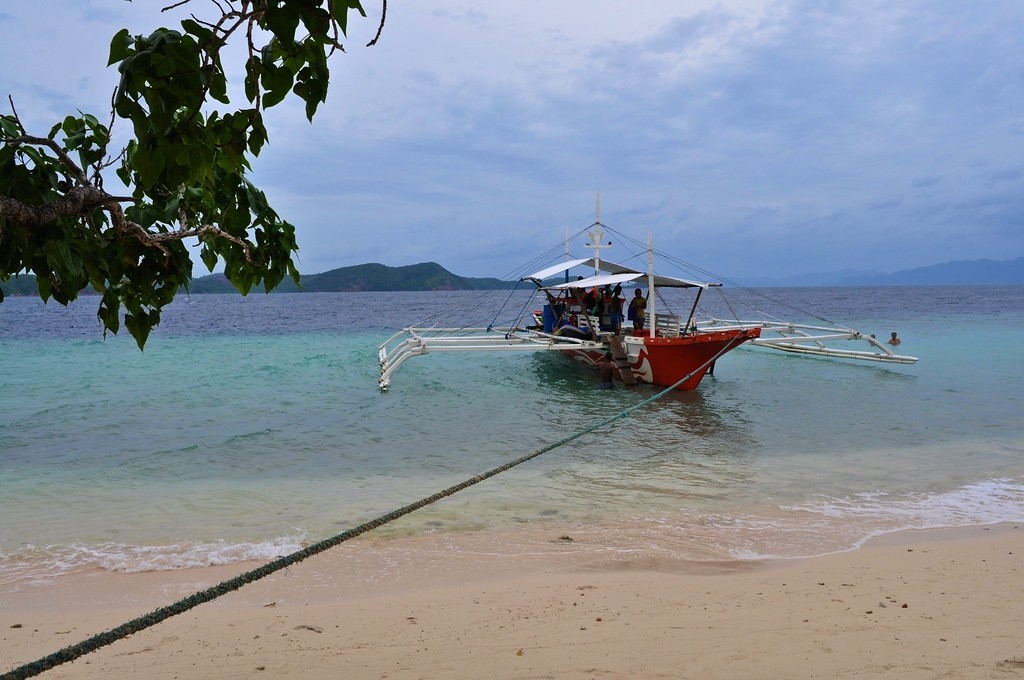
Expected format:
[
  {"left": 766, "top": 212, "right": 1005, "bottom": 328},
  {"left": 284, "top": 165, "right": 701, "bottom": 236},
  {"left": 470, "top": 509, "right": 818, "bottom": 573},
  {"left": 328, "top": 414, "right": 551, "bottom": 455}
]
[
  {"left": 487, "top": 256, "right": 680, "bottom": 340},
  {"left": 509, "top": 274, "right": 746, "bottom": 345}
]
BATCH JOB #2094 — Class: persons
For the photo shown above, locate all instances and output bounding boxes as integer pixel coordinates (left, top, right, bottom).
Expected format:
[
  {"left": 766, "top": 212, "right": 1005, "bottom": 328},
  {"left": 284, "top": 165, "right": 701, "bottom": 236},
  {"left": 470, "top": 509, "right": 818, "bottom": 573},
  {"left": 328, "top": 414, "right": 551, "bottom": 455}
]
[
  {"left": 576, "top": 275, "right": 647, "bottom": 335},
  {"left": 593, "top": 352, "right": 622, "bottom": 390},
  {"left": 869, "top": 332, "right": 900, "bottom": 345}
]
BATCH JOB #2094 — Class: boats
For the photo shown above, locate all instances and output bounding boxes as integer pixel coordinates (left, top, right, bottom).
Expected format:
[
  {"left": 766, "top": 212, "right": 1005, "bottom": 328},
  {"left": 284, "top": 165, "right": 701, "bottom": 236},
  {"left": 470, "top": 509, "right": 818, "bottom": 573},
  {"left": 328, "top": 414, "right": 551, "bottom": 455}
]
[{"left": 375, "top": 186, "right": 920, "bottom": 394}]
